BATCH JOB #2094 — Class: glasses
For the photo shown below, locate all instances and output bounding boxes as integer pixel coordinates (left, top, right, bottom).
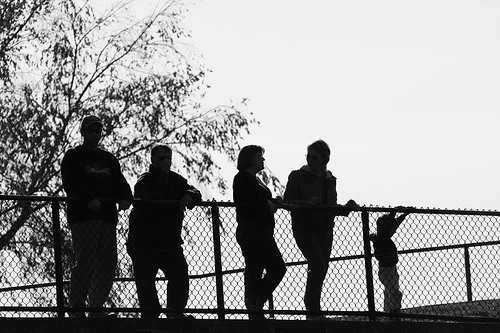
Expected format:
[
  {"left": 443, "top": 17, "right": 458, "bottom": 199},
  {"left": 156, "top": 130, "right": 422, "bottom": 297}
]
[
  {"left": 156, "top": 157, "right": 172, "bottom": 161},
  {"left": 307, "top": 154, "right": 322, "bottom": 160},
  {"left": 84, "top": 128, "right": 102, "bottom": 133}
]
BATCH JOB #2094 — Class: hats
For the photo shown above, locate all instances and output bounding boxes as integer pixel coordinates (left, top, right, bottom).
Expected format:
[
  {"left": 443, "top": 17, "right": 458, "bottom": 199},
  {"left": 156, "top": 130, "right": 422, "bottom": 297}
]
[{"left": 82, "top": 115, "right": 103, "bottom": 130}]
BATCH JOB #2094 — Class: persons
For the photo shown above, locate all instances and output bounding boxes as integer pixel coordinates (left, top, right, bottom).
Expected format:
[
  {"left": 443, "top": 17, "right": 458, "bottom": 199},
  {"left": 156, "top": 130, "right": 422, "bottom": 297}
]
[
  {"left": 283, "top": 139, "right": 359, "bottom": 321},
  {"left": 369, "top": 206, "right": 412, "bottom": 321},
  {"left": 60, "top": 115, "right": 133, "bottom": 321},
  {"left": 126, "top": 146, "right": 202, "bottom": 321},
  {"left": 232, "top": 145, "right": 287, "bottom": 321}
]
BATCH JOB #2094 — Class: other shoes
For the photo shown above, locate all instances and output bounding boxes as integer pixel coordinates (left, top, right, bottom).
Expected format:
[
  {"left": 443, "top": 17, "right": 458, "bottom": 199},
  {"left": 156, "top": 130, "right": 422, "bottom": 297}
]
[
  {"left": 306, "top": 315, "right": 332, "bottom": 320},
  {"left": 68, "top": 312, "right": 87, "bottom": 318},
  {"left": 249, "top": 314, "right": 276, "bottom": 320},
  {"left": 88, "top": 311, "right": 117, "bottom": 319},
  {"left": 166, "top": 313, "right": 196, "bottom": 319}
]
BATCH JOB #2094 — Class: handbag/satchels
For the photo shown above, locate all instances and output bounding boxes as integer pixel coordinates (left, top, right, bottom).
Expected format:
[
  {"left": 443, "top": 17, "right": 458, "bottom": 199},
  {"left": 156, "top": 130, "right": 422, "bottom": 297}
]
[{"left": 87, "top": 162, "right": 114, "bottom": 198}]
